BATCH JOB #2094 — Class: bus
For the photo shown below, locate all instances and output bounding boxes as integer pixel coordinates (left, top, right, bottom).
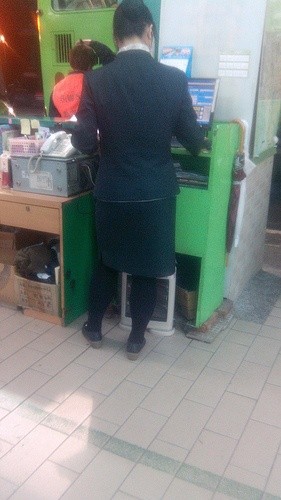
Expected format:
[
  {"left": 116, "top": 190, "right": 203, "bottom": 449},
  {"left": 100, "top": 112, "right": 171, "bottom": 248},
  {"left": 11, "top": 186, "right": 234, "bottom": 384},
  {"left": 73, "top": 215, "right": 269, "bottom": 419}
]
[{"left": 37, "top": 0, "right": 123, "bottom": 118}]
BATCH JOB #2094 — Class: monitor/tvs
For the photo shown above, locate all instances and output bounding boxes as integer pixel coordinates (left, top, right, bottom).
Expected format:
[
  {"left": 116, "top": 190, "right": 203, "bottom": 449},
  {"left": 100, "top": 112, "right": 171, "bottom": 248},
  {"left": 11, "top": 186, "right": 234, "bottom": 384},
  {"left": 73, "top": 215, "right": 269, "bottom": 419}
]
[{"left": 187, "top": 77, "right": 219, "bottom": 114}]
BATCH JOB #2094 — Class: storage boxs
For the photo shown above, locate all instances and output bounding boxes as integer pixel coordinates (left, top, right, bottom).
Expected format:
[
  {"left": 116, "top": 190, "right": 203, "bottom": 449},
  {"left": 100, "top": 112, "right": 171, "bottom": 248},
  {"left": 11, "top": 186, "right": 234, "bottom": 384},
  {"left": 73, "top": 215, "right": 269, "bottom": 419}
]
[
  {"left": 0, "top": 229, "right": 61, "bottom": 317},
  {"left": 177, "top": 283, "right": 196, "bottom": 321}
]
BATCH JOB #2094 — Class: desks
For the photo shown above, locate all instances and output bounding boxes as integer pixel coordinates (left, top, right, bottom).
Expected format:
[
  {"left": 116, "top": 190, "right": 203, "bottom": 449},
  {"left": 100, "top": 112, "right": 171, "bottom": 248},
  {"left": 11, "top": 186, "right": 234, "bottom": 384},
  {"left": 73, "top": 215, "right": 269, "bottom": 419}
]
[{"left": 0, "top": 190, "right": 94, "bottom": 328}]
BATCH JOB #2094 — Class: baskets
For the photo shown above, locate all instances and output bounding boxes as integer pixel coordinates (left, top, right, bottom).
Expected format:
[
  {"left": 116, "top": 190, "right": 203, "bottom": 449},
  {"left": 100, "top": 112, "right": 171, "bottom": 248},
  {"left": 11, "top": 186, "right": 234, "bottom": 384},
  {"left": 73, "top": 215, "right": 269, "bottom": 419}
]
[{"left": 9, "top": 136, "right": 45, "bottom": 152}]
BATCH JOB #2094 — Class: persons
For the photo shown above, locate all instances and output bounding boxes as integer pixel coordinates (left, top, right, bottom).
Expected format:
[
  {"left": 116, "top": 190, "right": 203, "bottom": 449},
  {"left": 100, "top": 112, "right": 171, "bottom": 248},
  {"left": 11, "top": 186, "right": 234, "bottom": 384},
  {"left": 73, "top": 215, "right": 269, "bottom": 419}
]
[
  {"left": 49, "top": 40, "right": 115, "bottom": 119},
  {"left": 71, "top": 0, "right": 205, "bottom": 359}
]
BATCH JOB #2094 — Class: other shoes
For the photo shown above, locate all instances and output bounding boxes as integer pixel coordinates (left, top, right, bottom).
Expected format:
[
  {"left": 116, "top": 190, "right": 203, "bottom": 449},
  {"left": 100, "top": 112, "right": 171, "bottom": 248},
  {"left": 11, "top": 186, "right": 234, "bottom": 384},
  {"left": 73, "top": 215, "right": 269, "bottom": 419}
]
[
  {"left": 83, "top": 321, "right": 102, "bottom": 348},
  {"left": 127, "top": 337, "right": 146, "bottom": 360}
]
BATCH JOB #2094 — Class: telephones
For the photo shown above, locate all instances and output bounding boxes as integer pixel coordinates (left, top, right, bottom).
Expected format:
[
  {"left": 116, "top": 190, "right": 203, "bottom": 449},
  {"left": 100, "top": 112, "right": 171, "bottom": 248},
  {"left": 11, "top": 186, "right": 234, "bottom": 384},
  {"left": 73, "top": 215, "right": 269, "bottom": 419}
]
[{"left": 38, "top": 130, "right": 79, "bottom": 159}]
